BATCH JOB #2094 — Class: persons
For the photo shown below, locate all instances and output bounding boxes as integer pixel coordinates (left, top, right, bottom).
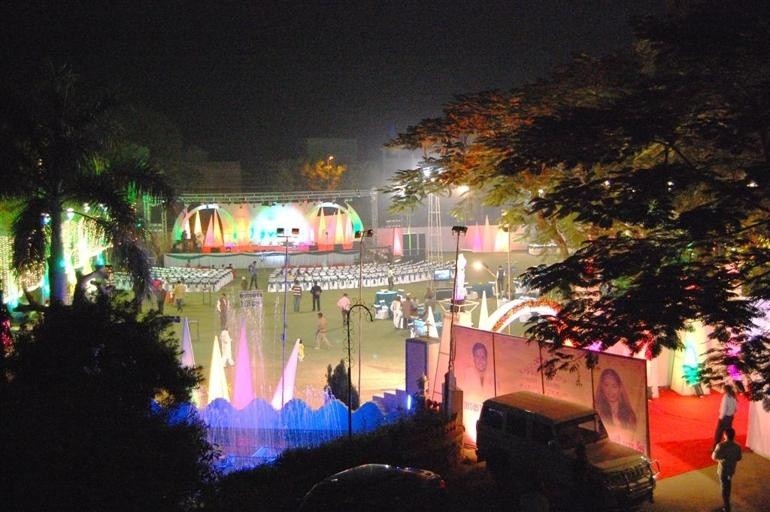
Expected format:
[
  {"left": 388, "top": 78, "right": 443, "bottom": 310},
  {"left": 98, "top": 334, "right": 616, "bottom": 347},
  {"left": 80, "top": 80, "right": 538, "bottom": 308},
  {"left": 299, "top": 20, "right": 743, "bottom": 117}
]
[
  {"left": 89, "top": 229, "right": 351, "bottom": 369},
  {"left": 495, "top": 266, "right": 506, "bottom": 296},
  {"left": 711, "top": 427, "right": 741, "bottom": 512},
  {"left": 572, "top": 443, "right": 593, "bottom": 504},
  {"left": 385, "top": 264, "right": 472, "bottom": 341},
  {"left": 153, "top": 385, "right": 209, "bottom": 437},
  {"left": 595, "top": 368, "right": 638, "bottom": 446},
  {"left": 708, "top": 386, "right": 737, "bottom": 454},
  {"left": 467, "top": 342, "right": 500, "bottom": 403}
]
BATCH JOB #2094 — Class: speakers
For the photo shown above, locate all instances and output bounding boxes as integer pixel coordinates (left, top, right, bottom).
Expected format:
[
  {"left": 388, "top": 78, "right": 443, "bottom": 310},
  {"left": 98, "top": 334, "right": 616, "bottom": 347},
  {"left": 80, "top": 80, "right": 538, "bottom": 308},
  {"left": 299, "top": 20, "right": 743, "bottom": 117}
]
[
  {"left": 352, "top": 241, "right": 360, "bottom": 248},
  {"left": 170, "top": 248, "right": 179, "bottom": 253},
  {"left": 210, "top": 248, "right": 220, "bottom": 253},
  {"left": 192, "top": 248, "right": 200, "bottom": 253},
  {"left": 309, "top": 245, "right": 318, "bottom": 251},
  {"left": 334, "top": 244, "right": 343, "bottom": 250}
]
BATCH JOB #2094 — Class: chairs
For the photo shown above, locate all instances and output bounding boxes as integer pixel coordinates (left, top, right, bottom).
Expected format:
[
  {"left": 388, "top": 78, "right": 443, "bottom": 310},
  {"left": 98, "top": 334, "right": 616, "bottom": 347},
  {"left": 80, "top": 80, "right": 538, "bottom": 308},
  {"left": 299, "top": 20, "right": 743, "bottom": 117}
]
[
  {"left": 266, "top": 259, "right": 458, "bottom": 293},
  {"left": 113, "top": 266, "right": 233, "bottom": 293}
]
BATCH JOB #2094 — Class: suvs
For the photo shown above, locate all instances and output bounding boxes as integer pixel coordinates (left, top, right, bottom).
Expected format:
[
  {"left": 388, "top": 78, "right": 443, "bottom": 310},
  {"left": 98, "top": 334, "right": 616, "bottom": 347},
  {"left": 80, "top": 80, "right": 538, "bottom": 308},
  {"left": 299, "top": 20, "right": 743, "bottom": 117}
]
[{"left": 476, "top": 392, "right": 658, "bottom": 506}]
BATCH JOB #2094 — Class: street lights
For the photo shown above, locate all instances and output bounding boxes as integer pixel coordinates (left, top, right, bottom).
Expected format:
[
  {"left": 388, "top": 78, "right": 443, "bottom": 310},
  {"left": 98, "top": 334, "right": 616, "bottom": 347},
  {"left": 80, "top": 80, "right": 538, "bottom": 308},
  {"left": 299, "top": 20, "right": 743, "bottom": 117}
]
[
  {"left": 354, "top": 228, "right": 375, "bottom": 407},
  {"left": 443, "top": 224, "right": 471, "bottom": 424},
  {"left": 498, "top": 220, "right": 512, "bottom": 336},
  {"left": 276, "top": 225, "right": 300, "bottom": 411}
]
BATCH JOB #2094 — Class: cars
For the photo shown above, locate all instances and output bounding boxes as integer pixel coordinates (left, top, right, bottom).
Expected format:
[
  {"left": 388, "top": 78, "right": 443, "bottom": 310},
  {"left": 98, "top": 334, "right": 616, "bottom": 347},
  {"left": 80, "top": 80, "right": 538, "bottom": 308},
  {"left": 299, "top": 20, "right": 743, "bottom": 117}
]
[{"left": 293, "top": 461, "right": 457, "bottom": 510}]
